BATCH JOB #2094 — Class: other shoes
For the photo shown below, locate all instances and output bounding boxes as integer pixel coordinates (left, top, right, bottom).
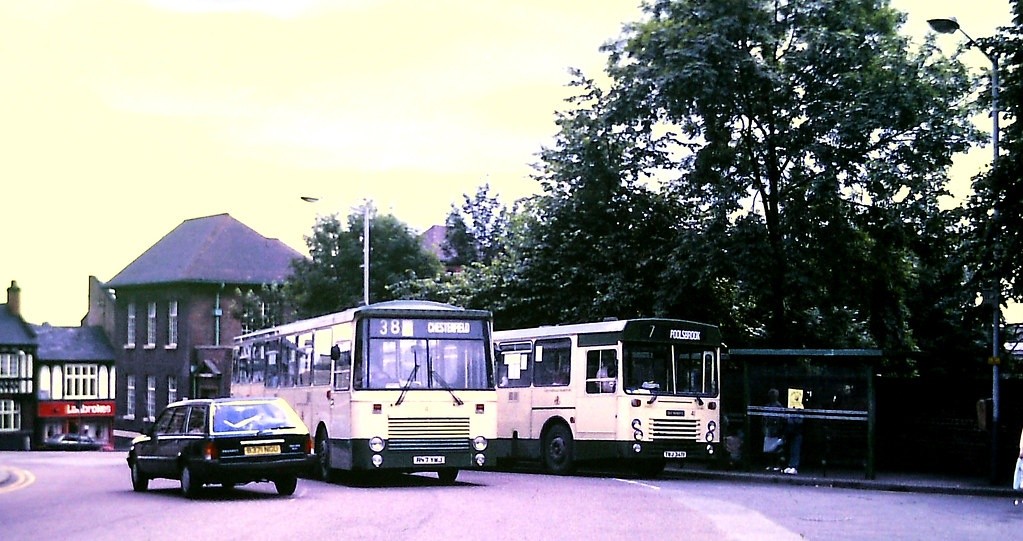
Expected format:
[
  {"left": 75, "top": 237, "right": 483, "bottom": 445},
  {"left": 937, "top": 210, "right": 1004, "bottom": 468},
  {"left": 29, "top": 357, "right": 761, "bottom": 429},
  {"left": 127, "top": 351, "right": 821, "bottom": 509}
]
[
  {"left": 784, "top": 467, "right": 797, "bottom": 474},
  {"left": 767, "top": 466, "right": 781, "bottom": 472}
]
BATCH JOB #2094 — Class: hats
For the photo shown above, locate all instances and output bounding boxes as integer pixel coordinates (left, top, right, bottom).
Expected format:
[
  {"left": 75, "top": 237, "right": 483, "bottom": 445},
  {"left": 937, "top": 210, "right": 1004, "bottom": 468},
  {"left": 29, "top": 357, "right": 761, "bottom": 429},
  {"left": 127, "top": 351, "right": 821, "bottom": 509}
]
[{"left": 769, "top": 389, "right": 779, "bottom": 396}]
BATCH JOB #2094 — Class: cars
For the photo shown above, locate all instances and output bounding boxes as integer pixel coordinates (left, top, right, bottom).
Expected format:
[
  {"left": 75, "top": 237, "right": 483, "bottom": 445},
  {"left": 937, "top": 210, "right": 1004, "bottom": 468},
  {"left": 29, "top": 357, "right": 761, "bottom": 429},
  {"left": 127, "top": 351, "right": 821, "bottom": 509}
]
[
  {"left": 43, "top": 433, "right": 107, "bottom": 451},
  {"left": 127, "top": 396, "right": 317, "bottom": 498}
]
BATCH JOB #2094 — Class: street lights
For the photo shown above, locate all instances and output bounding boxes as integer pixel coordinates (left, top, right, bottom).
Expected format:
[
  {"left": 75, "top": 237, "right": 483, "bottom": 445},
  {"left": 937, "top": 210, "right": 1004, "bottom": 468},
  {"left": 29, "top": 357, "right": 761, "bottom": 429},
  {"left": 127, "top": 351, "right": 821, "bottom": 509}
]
[
  {"left": 300, "top": 193, "right": 372, "bottom": 307},
  {"left": 925, "top": 17, "right": 1004, "bottom": 432}
]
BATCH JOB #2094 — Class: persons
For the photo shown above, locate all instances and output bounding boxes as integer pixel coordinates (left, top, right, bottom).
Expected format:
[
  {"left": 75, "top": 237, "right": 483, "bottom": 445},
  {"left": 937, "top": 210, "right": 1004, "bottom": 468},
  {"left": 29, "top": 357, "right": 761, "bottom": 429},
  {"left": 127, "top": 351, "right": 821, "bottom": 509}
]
[
  {"left": 763, "top": 388, "right": 806, "bottom": 475},
  {"left": 1019, "top": 429, "right": 1023, "bottom": 459},
  {"left": 593, "top": 355, "right": 616, "bottom": 392}
]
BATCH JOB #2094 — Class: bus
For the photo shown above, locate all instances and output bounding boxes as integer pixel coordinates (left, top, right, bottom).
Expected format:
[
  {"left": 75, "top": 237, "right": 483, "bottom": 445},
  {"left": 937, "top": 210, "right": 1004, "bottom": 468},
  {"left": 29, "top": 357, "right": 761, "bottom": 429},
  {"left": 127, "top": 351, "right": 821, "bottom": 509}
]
[
  {"left": 378, "top": 315, "right": 735, "bottom": 480},
  {"left": 226, "top": 301, "right": 498, "bottom": 485}
]
